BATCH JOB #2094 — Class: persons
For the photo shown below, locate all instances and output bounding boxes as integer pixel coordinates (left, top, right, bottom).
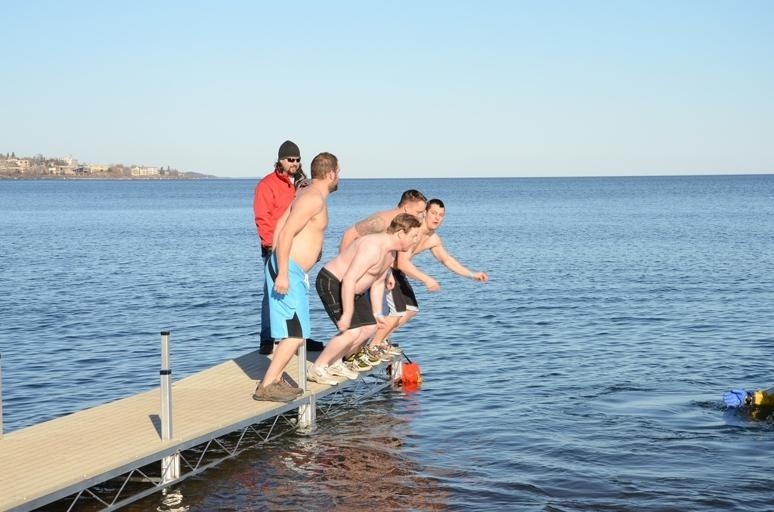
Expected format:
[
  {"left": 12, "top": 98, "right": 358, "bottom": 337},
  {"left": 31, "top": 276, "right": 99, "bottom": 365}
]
[
  {"left": 253, "top": 139, "right": 324, "bottom": 355},
  {"left": 342, "top": 190, "right": 429, "bottom": 371},
  {"left": 721, "top": 388, "right": 774, "bottom": 410},
  {"left": 253, "top": 152, "right": 340, "bottom": 400},
  {"left": 306, "top": 212, "right": 423, "bottom": 387},
  {"left": 369, "top": 199, "right": 490, "bottom": 362}
]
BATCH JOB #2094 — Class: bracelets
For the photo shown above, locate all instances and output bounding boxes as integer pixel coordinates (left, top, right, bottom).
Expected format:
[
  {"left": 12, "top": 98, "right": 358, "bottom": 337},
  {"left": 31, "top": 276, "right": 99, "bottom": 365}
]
[{"left": 470, "top": 271, "right": 474, "bottom": 279}]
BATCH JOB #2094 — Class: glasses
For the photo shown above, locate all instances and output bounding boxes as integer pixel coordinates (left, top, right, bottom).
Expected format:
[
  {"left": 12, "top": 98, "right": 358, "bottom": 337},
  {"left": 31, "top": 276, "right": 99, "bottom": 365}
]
[{"left": 285, "top": 157, "right": 301, "bottom": 162}]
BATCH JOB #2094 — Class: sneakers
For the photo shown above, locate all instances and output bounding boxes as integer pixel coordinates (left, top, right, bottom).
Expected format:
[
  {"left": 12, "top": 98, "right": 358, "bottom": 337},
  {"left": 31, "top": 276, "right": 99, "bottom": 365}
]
[
  {"left": 255, "top": 376, "right": 303, "bottom": 402},
  {"left": 306, "top": 364, "right": 338, "bottom": 385},
  {"left": 328, "top": 361, "right": 358, "bottom": 379},
  {"left": 343, "top": 339, "right": 401, "bottom": 371}
]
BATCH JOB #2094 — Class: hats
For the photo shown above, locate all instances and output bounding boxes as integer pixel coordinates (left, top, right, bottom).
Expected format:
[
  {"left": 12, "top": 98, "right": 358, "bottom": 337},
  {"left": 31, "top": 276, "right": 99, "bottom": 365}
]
[{"left": 279, "top": 140, "right": 300, "bottom": 158}]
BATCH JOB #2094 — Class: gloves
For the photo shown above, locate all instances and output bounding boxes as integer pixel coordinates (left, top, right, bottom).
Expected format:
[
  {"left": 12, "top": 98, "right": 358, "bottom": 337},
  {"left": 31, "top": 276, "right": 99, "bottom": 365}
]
[{"left": 724, "top": 390, "right": 754, "bottom": 408}]
